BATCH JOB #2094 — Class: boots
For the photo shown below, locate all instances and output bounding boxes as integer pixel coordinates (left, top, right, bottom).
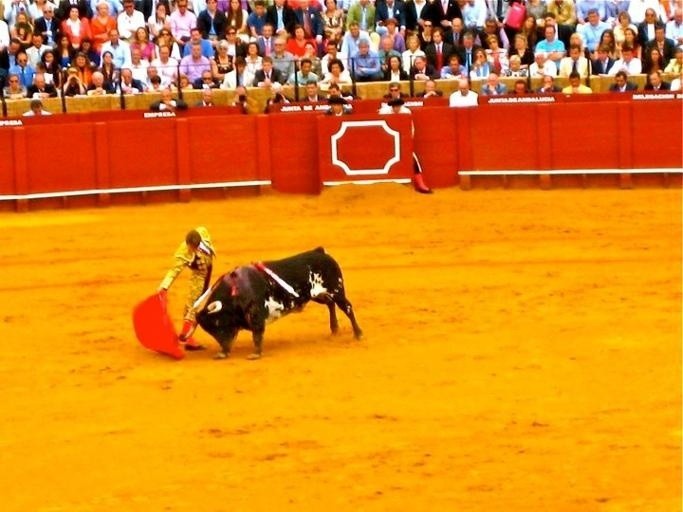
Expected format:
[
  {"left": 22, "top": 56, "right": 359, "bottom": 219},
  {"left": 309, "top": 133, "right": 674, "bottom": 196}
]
[
  {"left": 186, "top": 325, "right": 205, "bottom": 350},
  {"left": 179, "top": 320, "right": 194, "bottom": 343}
]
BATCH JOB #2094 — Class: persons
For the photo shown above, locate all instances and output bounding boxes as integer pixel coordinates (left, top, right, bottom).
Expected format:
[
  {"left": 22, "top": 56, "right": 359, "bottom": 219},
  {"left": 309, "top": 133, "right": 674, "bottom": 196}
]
[
  {"left": 157, "top": 225, "right": 217, "bottom": 353},
  {"left": 376, "top": 98, "right": 435, "bottom": 194},
  {"left": 1, "top": 0, "right": 683, "bottom": 117}
]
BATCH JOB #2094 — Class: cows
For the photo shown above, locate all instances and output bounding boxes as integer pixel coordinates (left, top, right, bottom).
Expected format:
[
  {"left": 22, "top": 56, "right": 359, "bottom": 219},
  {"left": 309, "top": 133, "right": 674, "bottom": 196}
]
[{"left": 196, "top": 246, "right": 364, "bottom": 360}]
